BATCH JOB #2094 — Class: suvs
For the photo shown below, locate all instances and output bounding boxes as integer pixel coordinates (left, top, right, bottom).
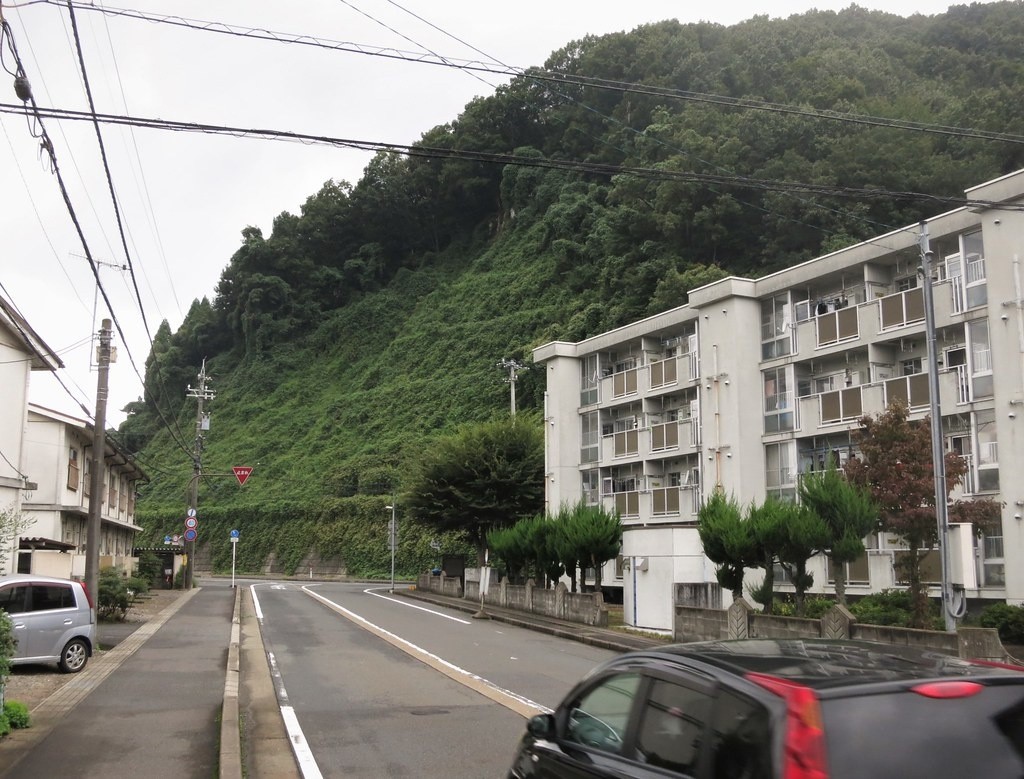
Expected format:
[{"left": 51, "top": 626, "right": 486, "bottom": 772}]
[{"left": 508, "top": 636, "right": 1024, "bottom": 779}]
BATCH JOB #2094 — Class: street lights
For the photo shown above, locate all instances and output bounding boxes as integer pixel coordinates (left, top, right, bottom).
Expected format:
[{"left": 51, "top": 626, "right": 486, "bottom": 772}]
[{"left": 386, "top": 503, "right": 394, "bottom": 595}]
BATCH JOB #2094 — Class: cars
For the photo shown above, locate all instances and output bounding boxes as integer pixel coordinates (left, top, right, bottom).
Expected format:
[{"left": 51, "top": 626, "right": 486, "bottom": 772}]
[{"left": 0, "top": 573, "right": 96, "bottom": 671}]
